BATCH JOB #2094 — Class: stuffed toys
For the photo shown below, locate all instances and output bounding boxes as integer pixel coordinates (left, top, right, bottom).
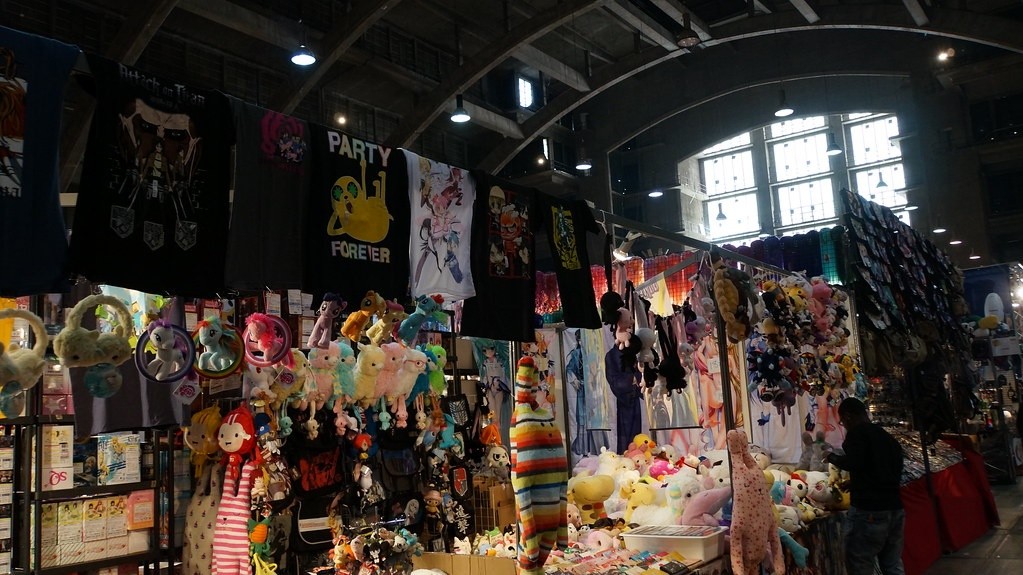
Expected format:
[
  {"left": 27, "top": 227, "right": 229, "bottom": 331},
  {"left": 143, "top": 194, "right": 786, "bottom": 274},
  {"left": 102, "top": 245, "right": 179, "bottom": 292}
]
[{"left": 0, "top": 258, "right": 873, "bottom": 575}]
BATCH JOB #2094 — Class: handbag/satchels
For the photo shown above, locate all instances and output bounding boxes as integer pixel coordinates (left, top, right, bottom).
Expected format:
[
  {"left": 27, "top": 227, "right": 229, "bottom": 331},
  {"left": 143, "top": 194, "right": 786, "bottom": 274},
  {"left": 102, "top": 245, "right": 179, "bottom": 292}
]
[{"left": 1013, "top": 436, "right": 1023, "bottom": 465}]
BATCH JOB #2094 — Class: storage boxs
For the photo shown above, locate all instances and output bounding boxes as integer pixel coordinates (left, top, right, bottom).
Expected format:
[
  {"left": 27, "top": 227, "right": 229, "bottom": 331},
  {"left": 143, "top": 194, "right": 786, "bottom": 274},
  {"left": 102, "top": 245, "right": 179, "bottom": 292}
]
[{"left": 618, "top": 522, "right": 730, "bottom": 562}]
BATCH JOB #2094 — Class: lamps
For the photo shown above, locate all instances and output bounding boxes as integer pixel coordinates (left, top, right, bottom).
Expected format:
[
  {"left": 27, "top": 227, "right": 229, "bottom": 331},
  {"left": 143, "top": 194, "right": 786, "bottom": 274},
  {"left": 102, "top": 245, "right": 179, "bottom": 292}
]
[
  {"left": 288, "top": 21, "right": 317, "bottom": 66},
  {"left": 450, "top": 94, "right": 471, "bottom": 123},
  {"left": 773, "top": 87, "right": 794, "bottom": 117},
  {"left": 675, "top": 12, "right": 700, "bottom": 48},
  {"left": 824, "top": 133, "right": 842, "bottom": 158},
  {"left": 575, "top": 147, "right": 593, "bottom": 171}
]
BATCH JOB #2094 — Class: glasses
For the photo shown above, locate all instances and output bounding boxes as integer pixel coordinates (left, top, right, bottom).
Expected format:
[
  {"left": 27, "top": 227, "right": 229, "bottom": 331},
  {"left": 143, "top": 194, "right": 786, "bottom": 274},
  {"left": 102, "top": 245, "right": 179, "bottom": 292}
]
[{"left": 839, "top": 414, "right": 846, "bottom": 426}]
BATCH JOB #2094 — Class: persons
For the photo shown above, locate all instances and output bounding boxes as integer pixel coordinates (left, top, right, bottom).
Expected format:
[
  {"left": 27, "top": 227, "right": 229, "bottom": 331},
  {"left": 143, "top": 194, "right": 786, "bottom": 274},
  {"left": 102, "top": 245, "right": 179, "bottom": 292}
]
[
  {"left": 822, "top": 398, "right": 905, "bottom": 575},
  {"left": 606, "top": 345, "right": 648, "bottom": 455}
]
